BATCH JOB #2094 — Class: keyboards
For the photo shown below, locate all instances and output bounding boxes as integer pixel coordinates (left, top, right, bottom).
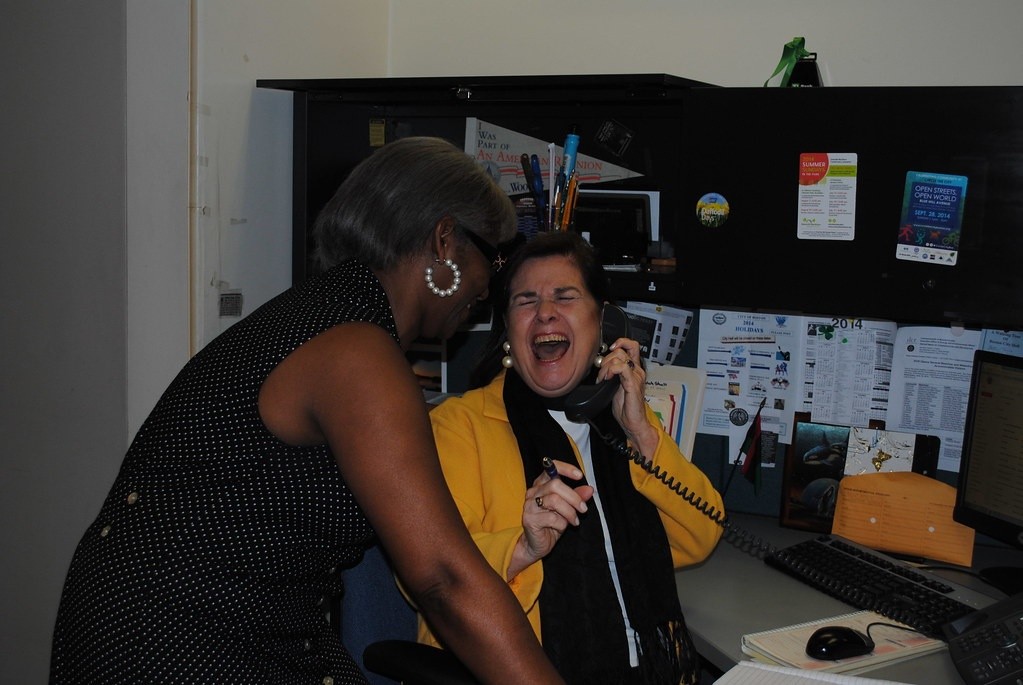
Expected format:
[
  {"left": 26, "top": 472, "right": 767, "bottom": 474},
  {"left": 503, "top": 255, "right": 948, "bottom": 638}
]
[{"left": 767, "top": 532, "right": 1001, "bottom": 644}]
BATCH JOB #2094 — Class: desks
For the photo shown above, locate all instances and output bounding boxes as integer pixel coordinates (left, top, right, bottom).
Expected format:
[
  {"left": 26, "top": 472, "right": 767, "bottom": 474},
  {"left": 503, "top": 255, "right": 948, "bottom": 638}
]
[{"left": 674, "top": 512, "right": 1023, "bottom": 685}]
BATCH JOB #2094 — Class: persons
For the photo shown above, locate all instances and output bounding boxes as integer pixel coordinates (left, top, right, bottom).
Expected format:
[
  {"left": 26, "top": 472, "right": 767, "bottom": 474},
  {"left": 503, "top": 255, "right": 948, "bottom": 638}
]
[
  {"left": 391, "top": 230, "right": 725, "bottom": 685},
  {"left": 51, "top": 137, "right": 568, "bottom": 683}
]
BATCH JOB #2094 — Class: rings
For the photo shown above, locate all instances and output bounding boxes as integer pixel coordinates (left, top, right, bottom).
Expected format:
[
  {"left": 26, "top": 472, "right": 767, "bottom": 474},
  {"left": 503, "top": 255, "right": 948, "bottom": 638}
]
[
  {"left": 535, "top": 497, "right": 547, "bottom": 510},
  {"left": 626, "top": 359, "right": 635, "bottom": 368}
]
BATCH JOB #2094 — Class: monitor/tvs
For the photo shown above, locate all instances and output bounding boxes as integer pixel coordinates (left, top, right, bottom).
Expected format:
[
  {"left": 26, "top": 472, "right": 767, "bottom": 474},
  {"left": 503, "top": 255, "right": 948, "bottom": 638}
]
[{"left": 953, "top": 349, "right": 1023, "bottom": 609}]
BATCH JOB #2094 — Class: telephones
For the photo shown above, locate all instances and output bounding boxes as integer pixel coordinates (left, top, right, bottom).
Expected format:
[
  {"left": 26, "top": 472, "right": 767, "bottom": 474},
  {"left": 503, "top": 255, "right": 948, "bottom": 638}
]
[{"left": 562, "top": 303, "right": 635, "bottom": 425}]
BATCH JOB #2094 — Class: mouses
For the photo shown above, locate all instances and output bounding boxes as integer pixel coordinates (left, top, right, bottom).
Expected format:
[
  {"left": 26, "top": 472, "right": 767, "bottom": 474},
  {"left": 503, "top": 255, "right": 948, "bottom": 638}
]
[{"left": 807, "top": 627, "right": 875, "bottom": 662}]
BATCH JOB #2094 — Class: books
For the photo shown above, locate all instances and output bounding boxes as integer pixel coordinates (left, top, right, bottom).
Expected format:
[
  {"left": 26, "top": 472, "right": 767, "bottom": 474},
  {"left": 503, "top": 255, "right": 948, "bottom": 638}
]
[
  {"left": 712, "top": 661, "right": 917, "bottom": 684},
  {"left": 741, "top": 604, "right": 949, "bottom": 679}
]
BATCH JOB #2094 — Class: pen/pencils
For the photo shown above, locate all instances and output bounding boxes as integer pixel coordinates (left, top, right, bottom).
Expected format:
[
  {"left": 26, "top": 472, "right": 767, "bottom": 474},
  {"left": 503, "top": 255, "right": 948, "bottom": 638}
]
[{"left": 519, "top": 133, "right": 580, "bottom": 233}]
